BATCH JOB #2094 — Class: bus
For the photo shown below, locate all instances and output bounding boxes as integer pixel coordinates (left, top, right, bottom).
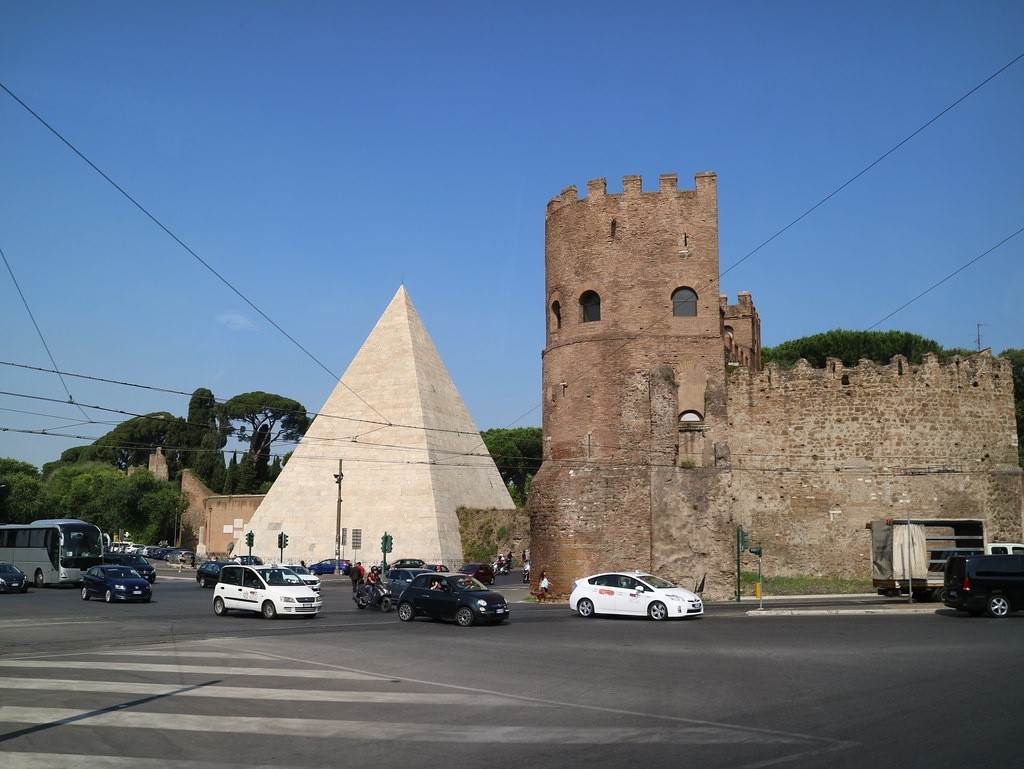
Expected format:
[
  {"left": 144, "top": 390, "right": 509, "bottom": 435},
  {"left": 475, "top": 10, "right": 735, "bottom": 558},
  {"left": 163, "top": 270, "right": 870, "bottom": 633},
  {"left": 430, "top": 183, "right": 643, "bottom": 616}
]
[
  {"left": 0, "top": 519, "right": 111, "bottom": 588},
  {"left": 105, "top": 542, "right": 133, "bottom": 553}
]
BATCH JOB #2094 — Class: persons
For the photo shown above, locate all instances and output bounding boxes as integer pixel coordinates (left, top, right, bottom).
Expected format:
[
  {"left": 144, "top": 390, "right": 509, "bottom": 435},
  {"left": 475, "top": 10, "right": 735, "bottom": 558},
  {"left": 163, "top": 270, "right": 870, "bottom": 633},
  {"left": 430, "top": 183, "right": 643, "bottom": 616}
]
[
  {"left": 349, "top": 562, "right": 365, "bottom": 592},
  {"left": 522, "top": 548, "right": 530, "bottom": 567},
  {"left": 457, "top": 578, "right": 476, "bottom": 589},
  {"left": 423, "top": 564, "right": 430, "bottom": 569},
  {"left": 435, "top": 565, "right": 442, "bottom": 572},
  {"left": 234, "top": 554, "right": 242, "bottom": 563},
  {"left": 539, "top": 570, "right": 554, "bottom": 593},
  {"left": 492, "top": 554, "right": 506, "bottom": 573},
  {"left": 301, "top": 560, "right": 306, "bottom": 568},
  {"left": 267, "top": 572, "right": 284, "bottom": 583},
  {"left": 389, "top": 564, "right": 397, "bottom": 570},
  {"left": 232, "top": 558, "right": 241, "bottom": 565},
  {"left": 363, "top": 565, "right": 383, "bottom": 604},
  {"left": 211, "top": 554, "right": 217, "bottom": 561},
  {"left": 506, "top": 551, "right": 514, "bottom": 562},
  {"left": 179, "top": 555, "right": 186, "bottom": 572},
  {"left": 190, "top": 553, "right": 195, "bottom": 569},
  {"left": 431, "top": 579, "right": 443, "bottom": 590}
]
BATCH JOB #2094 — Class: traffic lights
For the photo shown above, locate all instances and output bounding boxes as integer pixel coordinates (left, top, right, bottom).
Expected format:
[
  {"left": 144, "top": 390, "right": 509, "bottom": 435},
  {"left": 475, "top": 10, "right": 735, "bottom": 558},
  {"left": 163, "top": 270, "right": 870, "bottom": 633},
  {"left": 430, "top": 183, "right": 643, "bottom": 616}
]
[
  {"left": 246, "top": 533, "right": 253, "bottom": 547},
  {"left": 278, "top": 534, "right": 288, "bottom": 548}
]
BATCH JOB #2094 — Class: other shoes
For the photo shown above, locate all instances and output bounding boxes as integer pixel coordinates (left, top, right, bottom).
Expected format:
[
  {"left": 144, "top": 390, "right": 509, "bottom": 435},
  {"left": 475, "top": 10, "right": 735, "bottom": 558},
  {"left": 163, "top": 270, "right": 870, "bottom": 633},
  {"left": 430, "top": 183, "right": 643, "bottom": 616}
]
[{"left": 179, "top": 570, "right": 181, "bottom": 572}]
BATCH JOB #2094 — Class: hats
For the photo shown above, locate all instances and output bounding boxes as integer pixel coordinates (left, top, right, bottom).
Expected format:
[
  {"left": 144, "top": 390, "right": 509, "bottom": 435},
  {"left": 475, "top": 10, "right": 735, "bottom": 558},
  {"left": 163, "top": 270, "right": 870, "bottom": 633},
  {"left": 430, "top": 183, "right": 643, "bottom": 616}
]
[{"left": 356, "top": 562, "right": 361, "bottom": 565}]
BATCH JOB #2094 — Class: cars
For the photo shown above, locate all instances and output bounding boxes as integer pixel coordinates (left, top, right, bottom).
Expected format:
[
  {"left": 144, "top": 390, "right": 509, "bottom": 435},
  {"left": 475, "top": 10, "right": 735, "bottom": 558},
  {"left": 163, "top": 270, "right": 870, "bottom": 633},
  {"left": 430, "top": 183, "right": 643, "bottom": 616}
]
[
  {"left": 569, "top": 569, "right": 703, "bottom": 621},
  {"left": 457, "top": 563, "right": 495, "bottom": 585},
  {"left": 280, "top": 565, "right": 321, "bottom": 593},
  {"left": 212, "top": 565, "right": 322, "bottom": 620},
  {"left": 397, "top": 572, "right": 510, "bottom": 627},
  {"left": 195, "top": 560, "right": 242, "bottom": 588},
  {"left": 379, "top": 559, "right": 449, "bottom": 603},
  {"left": 125, "top": 544, "right": 195, "bottom": 563},
  {"left": 80, "top": 565, "right": 152, "bottom": 603},
  {"left": 0, "top": 563, "right": 29, "bottom": 593},
  {"left": 308, "top": 558, "right": 352, "bottom": 576}
]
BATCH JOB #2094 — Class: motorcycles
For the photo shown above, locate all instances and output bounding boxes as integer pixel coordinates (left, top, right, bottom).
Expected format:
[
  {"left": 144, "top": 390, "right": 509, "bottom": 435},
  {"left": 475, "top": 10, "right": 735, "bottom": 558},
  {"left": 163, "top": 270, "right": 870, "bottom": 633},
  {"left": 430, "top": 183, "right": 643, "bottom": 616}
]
[
  {"left": 521, "top": 560, "right": 531, "bottom": 583},
  {"left": 353, "top": 577, "right": 391, "bottom": 612},
  {"left": 492, "top": 562, "right": 510, "bottom": 575}
]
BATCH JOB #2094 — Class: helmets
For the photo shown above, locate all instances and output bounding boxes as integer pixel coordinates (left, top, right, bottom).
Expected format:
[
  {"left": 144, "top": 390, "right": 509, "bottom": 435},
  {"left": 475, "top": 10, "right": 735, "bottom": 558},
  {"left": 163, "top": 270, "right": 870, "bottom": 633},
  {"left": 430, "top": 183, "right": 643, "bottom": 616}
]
[
  {"left": 498, "top": 556, "right": 502, "bottom": 558},
  {"left": 371, "top": 566, "right": 378, "bottom": 571}
]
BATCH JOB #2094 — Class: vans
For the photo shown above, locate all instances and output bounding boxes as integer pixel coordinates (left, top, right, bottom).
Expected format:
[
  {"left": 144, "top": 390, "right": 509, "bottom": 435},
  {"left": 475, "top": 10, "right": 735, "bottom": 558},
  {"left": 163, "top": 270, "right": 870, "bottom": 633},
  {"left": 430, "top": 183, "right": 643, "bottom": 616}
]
[
  {"left": 943, "top": 554, "right": 1024, "bottom": 618},
  {"left": 104, "top": 553, "right": 156, "bottom": 583}
]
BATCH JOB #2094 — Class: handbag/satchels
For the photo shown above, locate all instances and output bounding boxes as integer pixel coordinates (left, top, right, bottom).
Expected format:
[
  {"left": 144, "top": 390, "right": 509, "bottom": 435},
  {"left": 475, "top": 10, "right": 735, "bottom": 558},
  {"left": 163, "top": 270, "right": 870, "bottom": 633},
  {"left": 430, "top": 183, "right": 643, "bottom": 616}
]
[{"left": 537, "top": 591, "right": 545, "bottom": 600}]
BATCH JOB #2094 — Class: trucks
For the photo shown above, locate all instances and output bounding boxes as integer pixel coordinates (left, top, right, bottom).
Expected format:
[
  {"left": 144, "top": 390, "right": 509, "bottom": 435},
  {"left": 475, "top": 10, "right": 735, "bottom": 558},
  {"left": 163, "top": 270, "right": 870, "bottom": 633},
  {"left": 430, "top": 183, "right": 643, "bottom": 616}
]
[{"left": 866, "top": 518, "right": 1024, "bottom": 601}]
